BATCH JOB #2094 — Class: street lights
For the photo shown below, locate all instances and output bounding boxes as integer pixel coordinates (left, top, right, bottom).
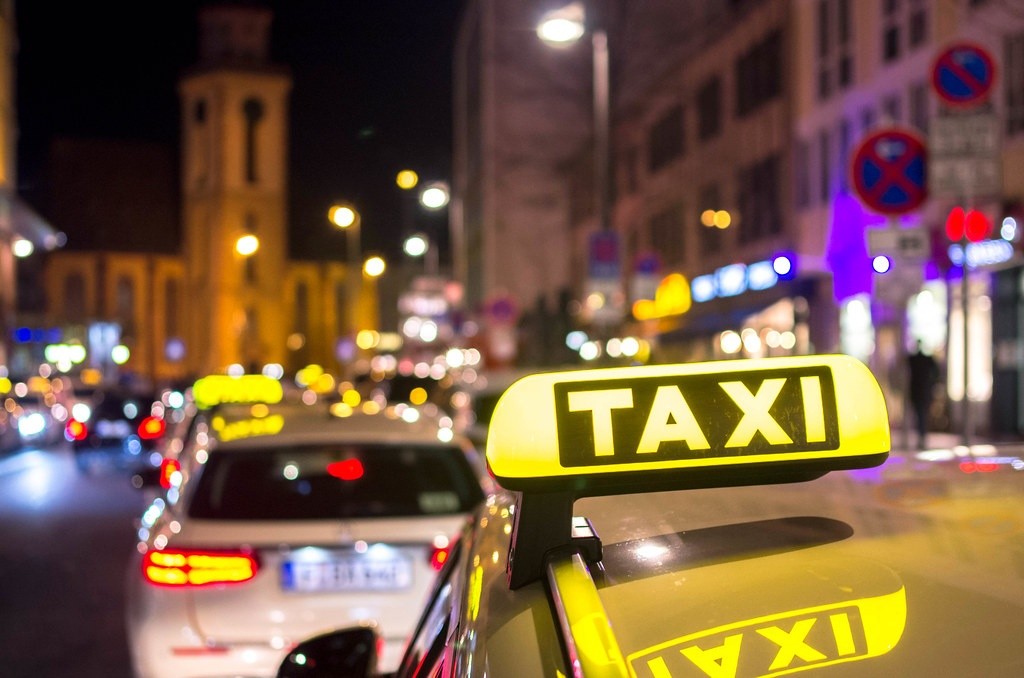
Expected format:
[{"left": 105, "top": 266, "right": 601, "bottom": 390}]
[{"left": 532, "top": 6, "right": 615, "bottom": 280}]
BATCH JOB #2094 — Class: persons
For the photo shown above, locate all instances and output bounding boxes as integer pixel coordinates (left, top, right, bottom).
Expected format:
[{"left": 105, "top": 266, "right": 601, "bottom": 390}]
[{"left": 906, "top": 338, "right": 941, "bottom": 452}]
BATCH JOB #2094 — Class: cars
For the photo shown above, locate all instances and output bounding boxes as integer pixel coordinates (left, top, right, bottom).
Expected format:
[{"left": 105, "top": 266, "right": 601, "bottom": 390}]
[
  {"left": 1, "top": 361, "right": 332, "bottom": 504},
  {"left": 120, "top": 403, "right": 503, "bottom": 678},
  {"left": 396, "top": 352, "right": 1024, "bottom": 678}
]
